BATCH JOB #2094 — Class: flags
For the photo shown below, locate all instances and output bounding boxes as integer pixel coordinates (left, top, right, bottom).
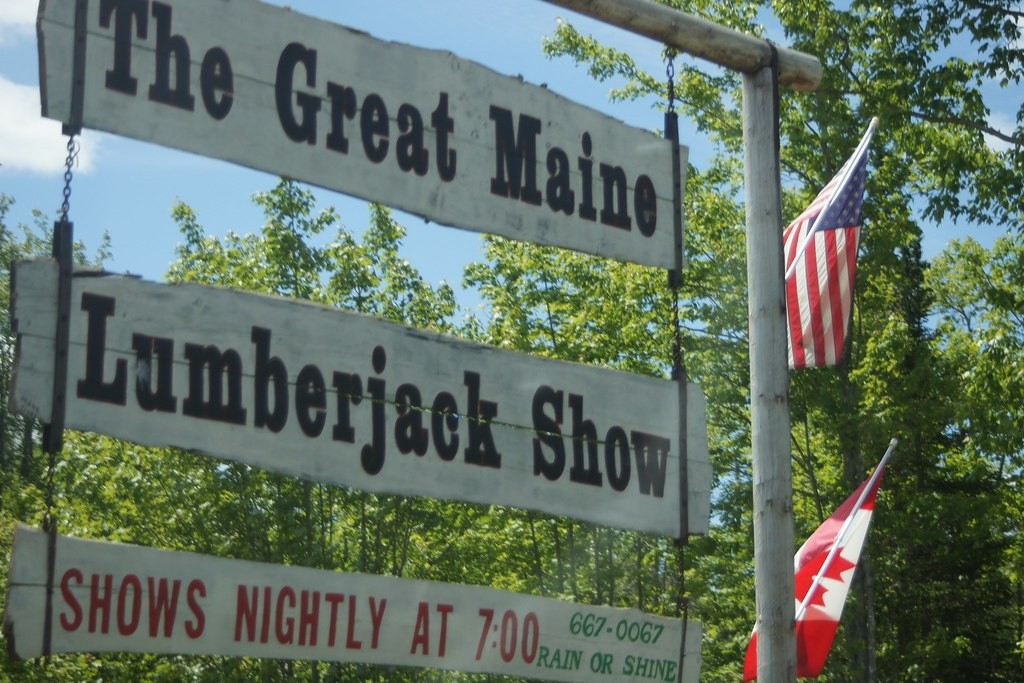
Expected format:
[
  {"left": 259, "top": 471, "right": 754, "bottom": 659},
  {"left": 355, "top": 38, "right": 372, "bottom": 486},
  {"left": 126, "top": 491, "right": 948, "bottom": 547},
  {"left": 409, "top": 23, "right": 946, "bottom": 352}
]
[
  {"left": 783, "top": 141, "right": 867, "bottom": 370},
  {"left": 744, "top": 464, "right": 887, "bottom": 683}
]
